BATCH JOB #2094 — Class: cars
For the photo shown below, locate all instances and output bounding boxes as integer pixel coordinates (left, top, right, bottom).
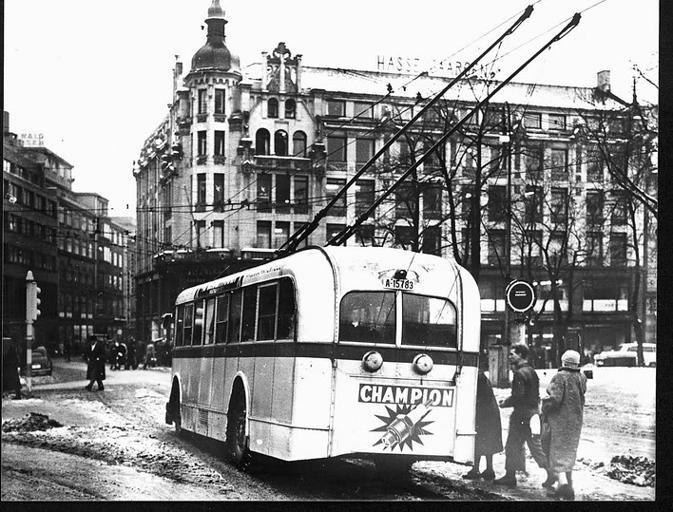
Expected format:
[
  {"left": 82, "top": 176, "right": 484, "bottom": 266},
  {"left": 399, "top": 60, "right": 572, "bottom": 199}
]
[{"left": 595, "top": 341, "right": 657, "bottom": 366}]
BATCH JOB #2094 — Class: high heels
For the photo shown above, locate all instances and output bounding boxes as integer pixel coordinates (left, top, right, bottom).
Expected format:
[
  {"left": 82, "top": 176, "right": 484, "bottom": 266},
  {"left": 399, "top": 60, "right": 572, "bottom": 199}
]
[{"left": 542, "top": 474, "right": 574, "bottom": 499}]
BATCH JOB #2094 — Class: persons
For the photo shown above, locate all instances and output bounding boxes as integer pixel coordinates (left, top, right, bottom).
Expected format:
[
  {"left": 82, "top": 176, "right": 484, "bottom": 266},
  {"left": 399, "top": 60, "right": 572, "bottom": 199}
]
[
  {"left": 62, "top": 336, "right": 71, "bottom": 361},
  {"left": 490, "top": 344, "right": 555, "bottom": 489},
  {"left": 539, "top": 349, "right": 587, "bottom": 500},
  {"left": 108, "top": 333, "right": 173, "bottom": 372},
  {"left": 79, "top": 334, "right": 106, "bottom": 391},
  {"left": 461, "top": 368, "right": 503, "bottom": 480}
]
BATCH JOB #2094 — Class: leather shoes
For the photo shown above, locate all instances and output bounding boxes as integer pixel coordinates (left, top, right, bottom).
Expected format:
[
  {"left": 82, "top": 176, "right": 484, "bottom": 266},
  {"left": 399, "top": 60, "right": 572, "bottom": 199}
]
[
  {"left": 494, "top": 474, "right": 516, "bottom": 486},
  {"left": 462, "top": 469, "right": 497, "bottom": 479}
]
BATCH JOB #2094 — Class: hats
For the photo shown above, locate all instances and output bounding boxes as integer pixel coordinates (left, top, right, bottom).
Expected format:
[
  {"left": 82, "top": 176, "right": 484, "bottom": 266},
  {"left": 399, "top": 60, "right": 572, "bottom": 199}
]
[{"left": 561, "top": 350, "right": 581, "bottom": 364}]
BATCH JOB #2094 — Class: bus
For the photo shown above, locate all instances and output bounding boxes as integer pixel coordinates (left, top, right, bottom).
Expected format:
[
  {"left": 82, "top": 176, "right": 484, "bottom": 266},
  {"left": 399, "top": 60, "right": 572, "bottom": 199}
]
[{"left": 166, "top": 245, "right": 481, "bottom": 474}]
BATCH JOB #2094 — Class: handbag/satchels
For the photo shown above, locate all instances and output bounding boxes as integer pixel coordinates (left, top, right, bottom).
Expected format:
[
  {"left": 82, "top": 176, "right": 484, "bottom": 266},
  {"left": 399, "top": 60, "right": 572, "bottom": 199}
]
[{"left": 541, "top": 424, "right": 551, "bottom": 453}]
